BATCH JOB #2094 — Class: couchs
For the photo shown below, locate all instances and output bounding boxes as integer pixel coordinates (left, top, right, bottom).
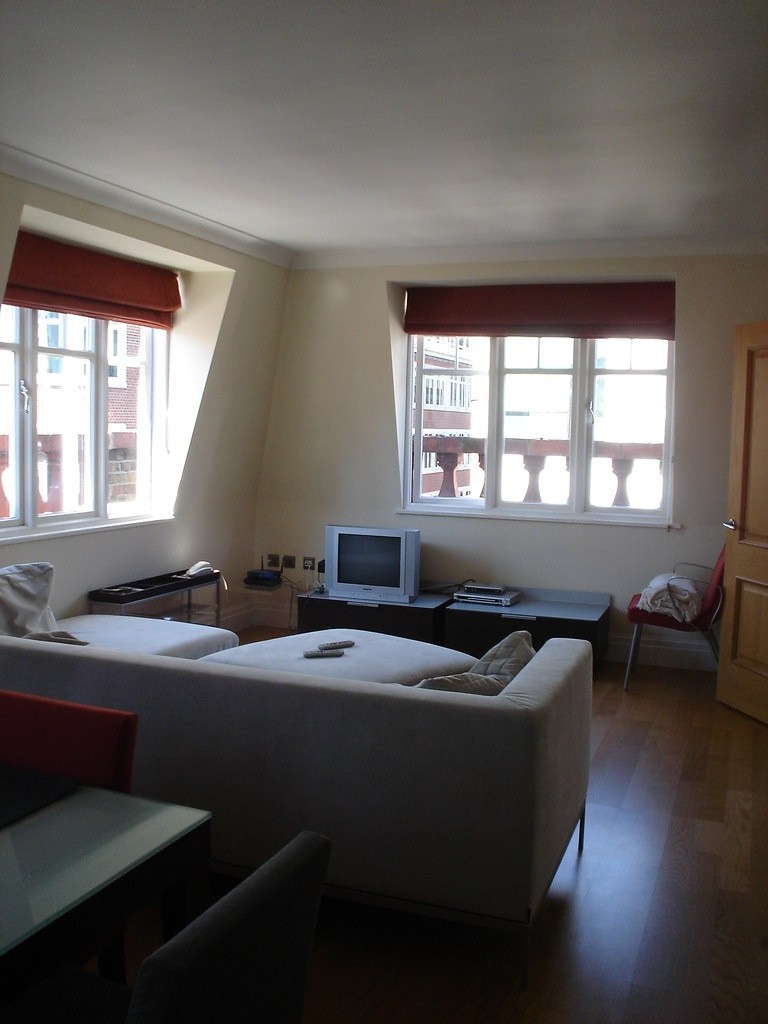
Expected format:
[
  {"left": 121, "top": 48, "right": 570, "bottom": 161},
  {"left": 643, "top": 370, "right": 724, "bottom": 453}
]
[{"left": 0, "top": 613, "right": 595, "bottom": 994}]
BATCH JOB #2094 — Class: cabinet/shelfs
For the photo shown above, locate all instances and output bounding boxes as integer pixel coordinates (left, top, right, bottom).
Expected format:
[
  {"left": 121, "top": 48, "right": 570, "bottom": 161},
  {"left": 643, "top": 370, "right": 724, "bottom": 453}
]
[
  {"left": 296, "top": 585, "right": 454, "bottom": 648},
  {"left": 443, "top": 597, "right": 608, "bottom": 683}
]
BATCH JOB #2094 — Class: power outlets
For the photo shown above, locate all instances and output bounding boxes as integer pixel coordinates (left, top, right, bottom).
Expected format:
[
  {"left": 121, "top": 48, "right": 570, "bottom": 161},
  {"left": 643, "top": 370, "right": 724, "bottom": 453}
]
[
  {"left": 282, "top": 555, "right": 295, "bottom": 569},
  {"left": 267, "top": 554, "right": 279, "bottom": 567},
  {"left": 303, "top": 556, "right": 315, "bottom": 571}
]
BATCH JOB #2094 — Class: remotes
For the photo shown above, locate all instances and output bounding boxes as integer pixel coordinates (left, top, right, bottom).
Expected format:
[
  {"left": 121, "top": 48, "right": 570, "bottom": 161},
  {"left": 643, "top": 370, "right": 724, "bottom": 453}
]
[
  {"left": 303, "top": 649, "right": 344, "bottom": 658},
  {"left": 316, "top": 640, "right": 355, "bottom": 649}
]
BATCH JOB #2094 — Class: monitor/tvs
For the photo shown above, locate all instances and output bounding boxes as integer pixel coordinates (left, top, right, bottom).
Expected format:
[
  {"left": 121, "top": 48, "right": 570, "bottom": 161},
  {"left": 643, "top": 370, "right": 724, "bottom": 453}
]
[{"left": 324, "top": 525, "right": 420, "bottom": 605}]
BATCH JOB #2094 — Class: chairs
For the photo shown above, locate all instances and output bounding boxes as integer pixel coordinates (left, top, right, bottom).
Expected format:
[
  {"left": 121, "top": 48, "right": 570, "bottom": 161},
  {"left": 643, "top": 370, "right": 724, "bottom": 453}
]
[
  {"left": 622, "top": 542, "right": 725, "bottom": 690},
  {"left": 0, "top": 828, "right": 331, "bottom": 1024}
]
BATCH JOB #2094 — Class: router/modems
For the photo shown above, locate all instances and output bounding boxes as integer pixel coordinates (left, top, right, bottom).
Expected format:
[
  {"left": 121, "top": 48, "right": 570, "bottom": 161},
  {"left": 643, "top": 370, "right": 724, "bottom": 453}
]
[{"left": 244, "top": 556, "right": 285, "bottom": 586}]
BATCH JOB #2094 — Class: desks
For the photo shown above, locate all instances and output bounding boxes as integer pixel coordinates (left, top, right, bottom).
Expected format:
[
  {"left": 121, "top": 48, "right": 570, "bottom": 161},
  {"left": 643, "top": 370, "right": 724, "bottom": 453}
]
[{"left": 0, "top": 786, "right": 212, "bottom": 974}]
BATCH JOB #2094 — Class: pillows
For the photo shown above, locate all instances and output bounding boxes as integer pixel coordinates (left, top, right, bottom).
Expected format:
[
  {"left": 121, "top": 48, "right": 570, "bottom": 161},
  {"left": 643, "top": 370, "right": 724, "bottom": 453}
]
[
  {"left": 410, "top": 629, "right": 534, "bottom": 694},
  {"left": 0, "top": 560, "right": 57, "bottom": 638}
]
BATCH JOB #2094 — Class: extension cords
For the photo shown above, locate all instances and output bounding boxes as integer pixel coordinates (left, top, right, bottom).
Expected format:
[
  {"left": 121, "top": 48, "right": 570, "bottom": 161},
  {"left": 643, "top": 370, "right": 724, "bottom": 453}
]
[{"left": 313, "top": 581, "right": 326, "bottom": 595}]
